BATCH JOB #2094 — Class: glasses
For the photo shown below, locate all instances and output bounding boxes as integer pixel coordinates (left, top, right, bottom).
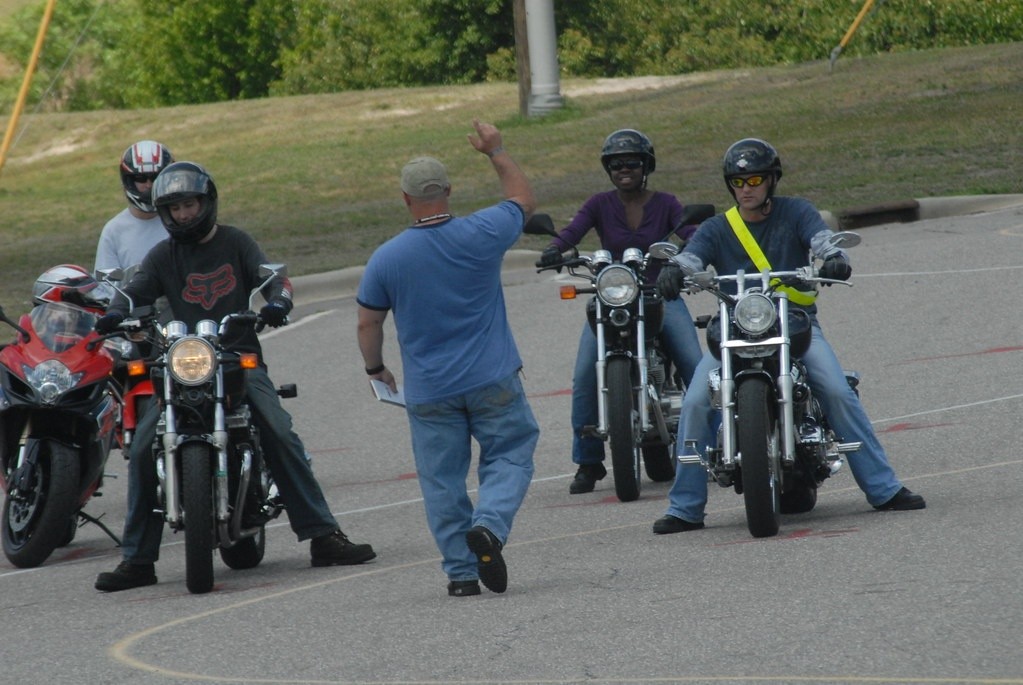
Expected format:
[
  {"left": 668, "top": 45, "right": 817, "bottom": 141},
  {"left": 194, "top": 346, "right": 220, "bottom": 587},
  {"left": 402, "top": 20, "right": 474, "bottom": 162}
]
[
  {"left": 132, "top": 175, "right": 158, "bottom": 183},
  {"left": 729, "top": 174, "right": 768, "bottom": 188},
  {"left": 608, "top": 157, "right": 645, "bottom": 170}
]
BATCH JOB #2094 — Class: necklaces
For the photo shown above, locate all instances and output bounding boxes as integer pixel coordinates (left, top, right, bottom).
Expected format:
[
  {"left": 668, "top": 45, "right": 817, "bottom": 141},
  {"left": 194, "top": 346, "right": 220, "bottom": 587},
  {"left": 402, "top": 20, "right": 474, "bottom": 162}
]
[{"left": 415, "top": 212, "right": 451, "bottom": 224}]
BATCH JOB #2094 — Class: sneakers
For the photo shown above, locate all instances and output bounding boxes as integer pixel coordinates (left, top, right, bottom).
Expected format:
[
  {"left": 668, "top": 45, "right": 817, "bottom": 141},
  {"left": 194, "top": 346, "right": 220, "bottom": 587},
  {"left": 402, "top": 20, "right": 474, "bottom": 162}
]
[
  {"left": 446, "top": 580, "right": 480, "bottom": 596},
  {"left": 311, "top": 530, "right": 376, "bottom": 566},
  {"left": 466, "top": 526, "right": 508, "bottom": 593},
  {"left": 95, "top": 563, "right": 157, "bottom": 591},
  {"left": 653, "top": 514, "right": 704, "bottom": 533},
  {"left": 875, "top": 487, "right": 926, "bottom": 510},
  {"left": 570, "top": 461, "right": 607, "bottom": 495}
]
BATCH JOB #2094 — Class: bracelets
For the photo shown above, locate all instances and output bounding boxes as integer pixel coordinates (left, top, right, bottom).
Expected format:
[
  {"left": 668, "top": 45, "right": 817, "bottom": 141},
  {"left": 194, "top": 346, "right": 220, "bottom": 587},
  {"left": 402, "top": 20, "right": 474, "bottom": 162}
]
[
  {"left": 489, "top": 145, "right": 504, "bottom": 157},
  {"left": 365, "top": 364, "right": 384, "bottom": 375}
]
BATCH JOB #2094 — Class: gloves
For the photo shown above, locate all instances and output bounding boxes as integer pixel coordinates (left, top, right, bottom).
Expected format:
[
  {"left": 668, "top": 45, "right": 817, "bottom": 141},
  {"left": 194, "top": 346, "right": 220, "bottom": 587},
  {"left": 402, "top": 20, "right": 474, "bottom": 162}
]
[
  {"left": 542, "top": 246, "right": 563, "bottom": 273},
  {"left": 96, "top": 312, "right": 123, "bottom": 335},
  {"left": 254, "top": 295, "right": 293, "bottom": 333},
  {"left": 655, "top": 264, "right": 685, "bottom": 302},
  {"left": 819, "top": 251, "right": 852, "bottom": 284}
]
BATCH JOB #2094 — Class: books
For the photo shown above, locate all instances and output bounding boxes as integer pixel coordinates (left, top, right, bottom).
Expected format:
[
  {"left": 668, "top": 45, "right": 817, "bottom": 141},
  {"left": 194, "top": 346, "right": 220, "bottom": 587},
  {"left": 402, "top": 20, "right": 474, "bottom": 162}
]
[{"left": 369, "top": 379, "right": 406, "bottom": 408}]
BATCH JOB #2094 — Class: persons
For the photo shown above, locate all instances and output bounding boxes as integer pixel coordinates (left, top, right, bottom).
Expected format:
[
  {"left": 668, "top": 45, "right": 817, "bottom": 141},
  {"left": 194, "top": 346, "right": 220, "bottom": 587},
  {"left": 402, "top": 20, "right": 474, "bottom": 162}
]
[
  {"left": 541, "top": 130, "right": 702, "bottom": 492},
  {"left": 653, "top": 139, "right": 925, "bottom": 535},
  {"left": 355, "top": 118, "right": 540, "bottom": 597},
  {"left": 93, "top": 161, "right": 376, "bottom": 590},
  {"left": 94, "top": 139, "right": 175, "bottom": 421}
]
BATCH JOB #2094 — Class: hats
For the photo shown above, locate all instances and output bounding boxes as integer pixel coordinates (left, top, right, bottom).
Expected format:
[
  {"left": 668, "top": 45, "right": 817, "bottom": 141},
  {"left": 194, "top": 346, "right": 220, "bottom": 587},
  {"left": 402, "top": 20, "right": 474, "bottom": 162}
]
[{"left": 401, "top": 157, "right": 447, "bottom": 196}]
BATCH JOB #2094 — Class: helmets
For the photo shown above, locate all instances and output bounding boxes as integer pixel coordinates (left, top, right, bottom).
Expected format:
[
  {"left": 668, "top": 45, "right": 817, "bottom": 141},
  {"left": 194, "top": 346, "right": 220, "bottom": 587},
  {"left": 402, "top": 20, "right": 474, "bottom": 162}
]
[
  {"left": 600, "top": 128, "right": 656, "bottom": 176},
  {"left": 33, "top": 264, "right": 110, "bottom": 309},
  {"left": 151, "top": 161, "right": 217, "bottom": 244},
  {"left": 724, "top": 138, "right": 782, "bottom": 195},
  {"left": 120, "top": 140, "right": 174, "bottom": 212}
]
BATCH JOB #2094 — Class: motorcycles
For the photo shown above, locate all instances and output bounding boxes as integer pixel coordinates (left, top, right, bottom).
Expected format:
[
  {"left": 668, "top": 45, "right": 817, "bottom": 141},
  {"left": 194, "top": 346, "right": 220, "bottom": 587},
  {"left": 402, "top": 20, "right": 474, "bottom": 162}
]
[
  {"left": 0, "top": 263, "right": 313, "bottom": 594},
  {"left": 654, "top": 231, "right": 863, "bottom": 538},
  {"left": 522, "top": 212, "right": 696, "bottom": 502}
]
